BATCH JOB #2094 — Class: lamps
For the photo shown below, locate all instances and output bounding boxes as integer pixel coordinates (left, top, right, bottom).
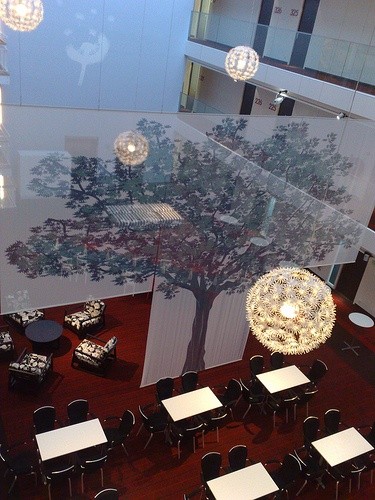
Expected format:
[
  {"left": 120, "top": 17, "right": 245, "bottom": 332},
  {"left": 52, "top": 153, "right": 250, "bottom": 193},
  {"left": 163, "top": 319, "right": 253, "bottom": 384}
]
[
  {"left": 0, "top": 0, "right": 44, "bottom": 32},
  {"left": 112, "top": 129, "right": 148, "bottom": 166},
  {"left": 224, "top": 45, "right": 260, "bottom": 81},
  {"left": 336, "top": 112, "right": 346, "bottom": 120},
  {"left": 245, "top": 266, "right": 336, "bottom": 356}
]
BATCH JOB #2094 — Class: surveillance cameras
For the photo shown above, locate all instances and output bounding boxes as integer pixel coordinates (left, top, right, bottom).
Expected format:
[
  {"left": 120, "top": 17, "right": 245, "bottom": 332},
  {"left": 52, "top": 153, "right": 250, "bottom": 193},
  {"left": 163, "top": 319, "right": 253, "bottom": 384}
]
[{"left": 274, "top": 97, "right": 283, "bottom": 104}]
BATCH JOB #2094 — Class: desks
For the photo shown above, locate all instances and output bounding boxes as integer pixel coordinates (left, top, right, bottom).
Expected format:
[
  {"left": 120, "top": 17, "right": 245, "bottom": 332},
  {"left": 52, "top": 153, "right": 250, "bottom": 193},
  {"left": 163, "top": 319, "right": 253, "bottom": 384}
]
[
  {"left": 206, "top": 462, "right": 279, "bottom": 500},
  {"left": 256, "top": 364, "right": 311, "bottom": 416},
  {"left": 25, "top": 319, "right": 63, "bottom": 353},
  {"left": 160, "top": 386, "right": 224, "bottom": 446},
  {"left": 348, "top": 313, "right": 374, "bottom": 331},
  {"left": 310, "top": 427, "right": 374, "bottom": 491},
  {"left": 35, "top": 418, "right": 108, "bottom": 462}
]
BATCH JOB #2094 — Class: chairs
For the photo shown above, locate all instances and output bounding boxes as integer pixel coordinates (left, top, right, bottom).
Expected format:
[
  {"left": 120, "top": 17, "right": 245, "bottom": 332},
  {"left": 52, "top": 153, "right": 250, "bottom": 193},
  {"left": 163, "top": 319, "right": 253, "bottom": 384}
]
[{"left": 0, "top": 286, "right": 375, "bottom": 500}]
